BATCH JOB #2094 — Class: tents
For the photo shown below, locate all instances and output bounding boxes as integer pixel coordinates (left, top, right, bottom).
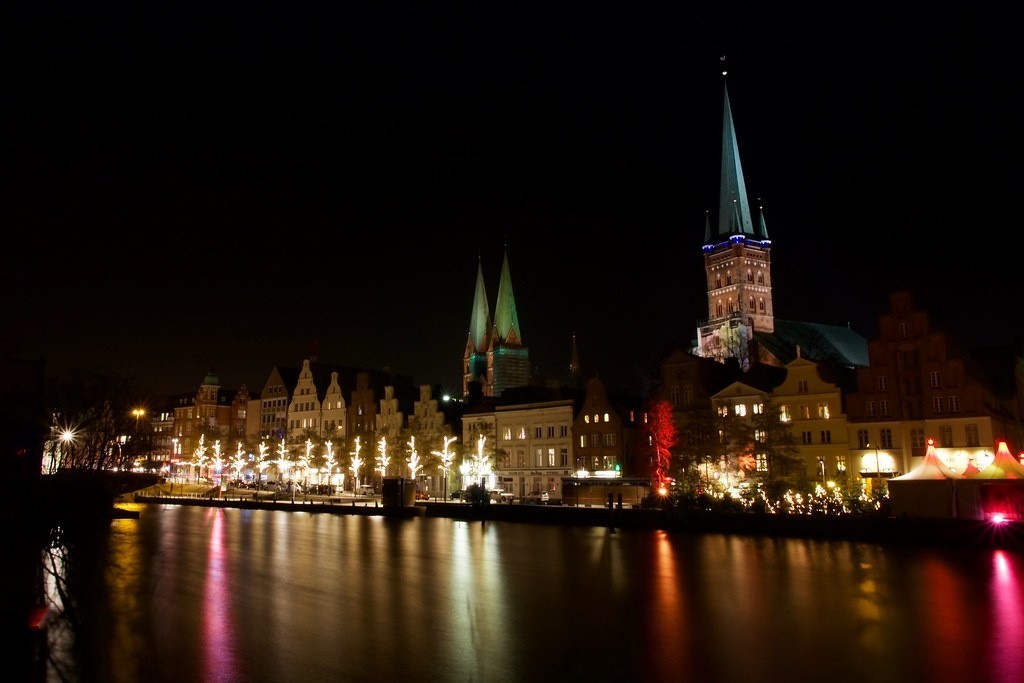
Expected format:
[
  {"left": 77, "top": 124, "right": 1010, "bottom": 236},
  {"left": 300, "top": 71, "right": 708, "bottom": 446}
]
[{"left": 886, "top": 442, "right": 1024, "bottom": 523}]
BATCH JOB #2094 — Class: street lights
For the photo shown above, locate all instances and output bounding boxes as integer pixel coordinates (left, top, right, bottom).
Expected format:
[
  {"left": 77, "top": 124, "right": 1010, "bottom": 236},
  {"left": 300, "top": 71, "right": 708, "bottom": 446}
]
[
  {"left": 191, "top": 432, "right": 208, "bottom": 483},
  {"left": 131, "top": 407, "right": 146, "bottom": 428},
  {"left": 58, "top": 428, "right": 73, "bottom": 449},
  {"left": 373, "top": 435, "right": 389, "bottom": 484},
  {"left": 297, "top": 434, "right": 313, "bottom": 496},
  {"left": 463, "top": 432, "right": 499, "bottom": 504},
  {"left": 276, "top": 437, "right": 288, "bottom": 497},
  {"left": 228, "top": 439, "right": 247, "bottom": 489},
  {"left": 323, "top": 439, "right": 339, "bottom": 498},
  {"left": 212, "top": 436, "right": 224, "bottom": 497},
  {"left": 405, "top": 436, "right": 421, "bottom": 478},
  {"left": 350, "top": 433, "right": 362, "bottom": 497},
  {"left": 254, "top": 438, "right": 272, "bottom": 495},
  {"left": 432, "top": 432, "right": 460, "bottom": 502}
]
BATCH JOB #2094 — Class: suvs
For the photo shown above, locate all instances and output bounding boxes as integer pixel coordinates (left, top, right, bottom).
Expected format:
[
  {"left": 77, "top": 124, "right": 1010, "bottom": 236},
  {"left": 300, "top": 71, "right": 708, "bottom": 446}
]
[{"left": 490, "top": 488, "right": 515, "bottom": 501}]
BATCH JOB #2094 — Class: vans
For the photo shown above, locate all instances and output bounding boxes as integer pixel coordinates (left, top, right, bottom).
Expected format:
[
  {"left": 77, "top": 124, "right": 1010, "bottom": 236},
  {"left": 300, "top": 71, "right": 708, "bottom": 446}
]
[{"left": 524, "top": 489, "right": 550, "bottom": 501}]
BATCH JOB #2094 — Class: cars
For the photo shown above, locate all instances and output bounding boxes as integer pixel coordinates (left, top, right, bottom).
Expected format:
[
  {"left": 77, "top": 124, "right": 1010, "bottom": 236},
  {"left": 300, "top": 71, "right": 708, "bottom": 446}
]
[
  {"left": 355, "top": 484, "right": 374, "bottom": 494},
  {"left": 265, "top": 480, "right": 286, "bottom": 492},
  {"left": 450, "top": 489, "right": 469, "bottom": 501},
  {"left": 308, "top": 484, "right": 335, "bottom": 496},
  {"left": 415, "top": 488, "right": 429, "bottom": 500}
]
